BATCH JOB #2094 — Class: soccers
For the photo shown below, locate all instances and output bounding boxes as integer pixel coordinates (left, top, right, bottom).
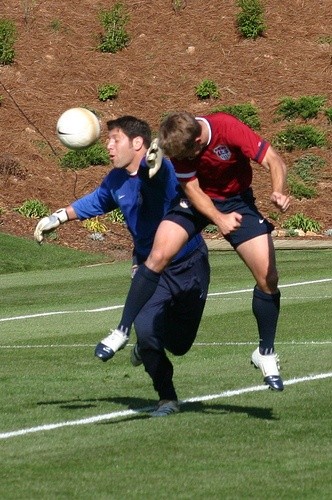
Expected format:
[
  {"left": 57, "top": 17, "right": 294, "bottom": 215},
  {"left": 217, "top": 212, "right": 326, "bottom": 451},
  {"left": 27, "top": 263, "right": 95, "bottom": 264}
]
[{"left": 56, "top": 107, "right": 100, "bottom": 150}]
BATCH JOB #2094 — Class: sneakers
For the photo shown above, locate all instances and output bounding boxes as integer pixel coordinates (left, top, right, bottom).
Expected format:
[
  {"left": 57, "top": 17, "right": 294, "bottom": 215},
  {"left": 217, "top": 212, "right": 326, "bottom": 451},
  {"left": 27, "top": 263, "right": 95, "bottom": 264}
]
[
  {"left": 131, "top": 343, "right": 142, "bottom": 366},
  {"left": 150, "top": 400, "right": 178, "bottom": 417},
  {"left": 94, "top": 328, "right": 130, "bottom": 362},
  {"left": 250, "top": 346, "right": 283, "bottom": 392}
]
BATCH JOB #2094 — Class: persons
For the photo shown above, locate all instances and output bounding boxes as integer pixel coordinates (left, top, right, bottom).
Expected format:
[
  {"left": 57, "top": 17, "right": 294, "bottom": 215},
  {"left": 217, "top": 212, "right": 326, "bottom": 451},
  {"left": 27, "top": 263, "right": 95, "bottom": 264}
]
[
  {"left": 94, "top": 112, "right": 292, "bottom": 392},
  {"left": 33, "top": 116, "right": 211, "bottom": 416}
]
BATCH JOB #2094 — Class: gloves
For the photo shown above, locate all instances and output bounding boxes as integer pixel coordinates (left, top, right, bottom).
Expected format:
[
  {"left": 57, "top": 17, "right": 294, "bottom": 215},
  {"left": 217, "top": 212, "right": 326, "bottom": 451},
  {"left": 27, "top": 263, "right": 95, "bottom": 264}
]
[
  {"left": 34, "top": 208, "right": 70, "bottom": 246},
  {"left": 145, "top": 137, "right": 163, "bottom": 178}
]
